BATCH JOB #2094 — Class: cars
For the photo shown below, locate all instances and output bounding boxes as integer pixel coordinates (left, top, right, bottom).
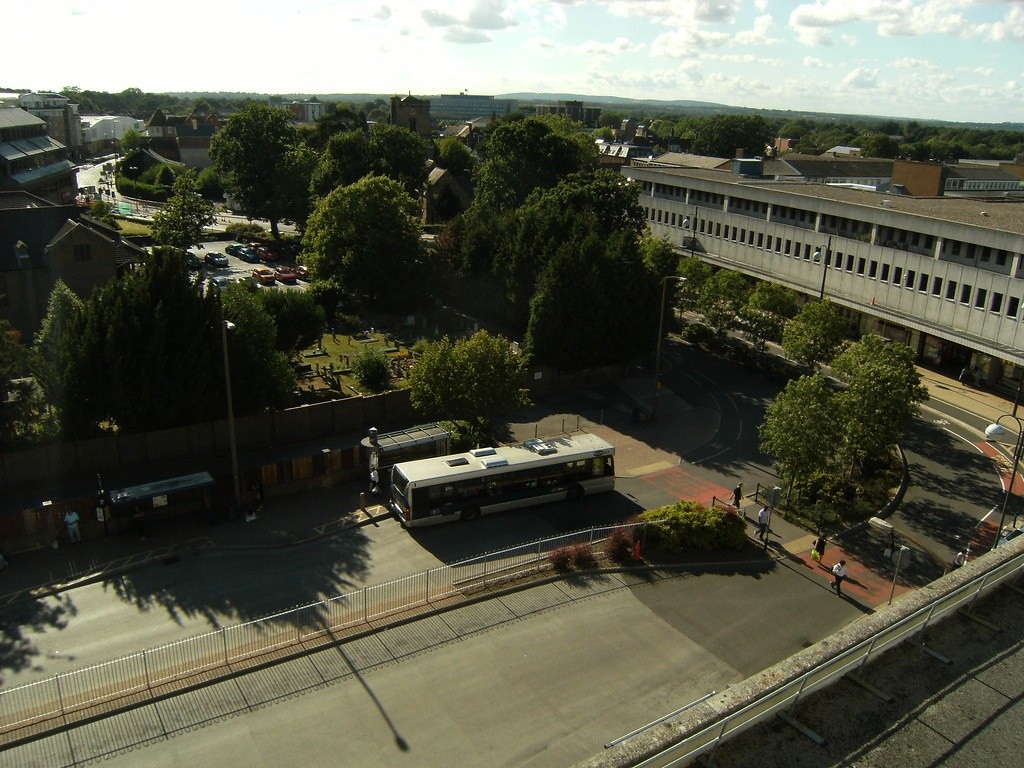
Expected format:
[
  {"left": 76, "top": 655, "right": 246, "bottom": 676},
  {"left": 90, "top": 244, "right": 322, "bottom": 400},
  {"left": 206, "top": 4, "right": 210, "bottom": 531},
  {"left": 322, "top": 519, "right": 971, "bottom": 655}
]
[
  {"left": 257, "top": 247, "right": 278, "bottom": 261},
  {"left": 183, "top": 252, "right": 200, "bottom": 267},
  {"left": 237, "top": 276, "right": 259, "bottom": 288},
  {"left": 247, "top": 242, "right": 262, "bottom": 252},
  {"left": 291, "top": 265, "right": 310, "bottom": 279},
  {"left": 236, "top": 246, "right": 256, "bottom": 261},
  {"left": 273, "top": 266, "right": 296, "bottom": 284},
  {"left": 206, "top": 251, "right": 228, "bottom": 267},
  {"left": 253, "top": 268, "right": 275, "bottom": 285},
  {"left": 224, "top": 244, "right": 244, "bottom": 256}
]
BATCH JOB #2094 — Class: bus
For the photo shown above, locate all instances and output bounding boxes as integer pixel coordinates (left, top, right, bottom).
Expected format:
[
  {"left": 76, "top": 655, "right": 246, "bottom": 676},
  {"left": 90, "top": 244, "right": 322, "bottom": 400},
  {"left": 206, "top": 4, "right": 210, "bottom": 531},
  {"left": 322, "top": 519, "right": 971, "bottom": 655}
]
[{"left": 386, "top": 430, "right": 617, "bottom": 529}]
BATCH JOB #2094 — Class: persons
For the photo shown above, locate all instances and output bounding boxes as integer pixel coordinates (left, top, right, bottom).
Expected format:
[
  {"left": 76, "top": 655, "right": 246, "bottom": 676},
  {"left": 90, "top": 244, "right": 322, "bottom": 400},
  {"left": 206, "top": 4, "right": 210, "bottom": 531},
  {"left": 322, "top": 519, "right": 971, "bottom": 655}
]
[
  {"left": 811, "top": 532, "right": 829, "bottom": 562},
  {"left": 753, "top": 505, "right": 770, "bottom": 542},
  {"left": 883, "top": 526, "right": 896, "bottom": 563},
  {"left": 368, "top": 466, "right": 379, "bottom": 497},
  {"left": 973, "top": 368, "right": 984, "bottom": 389},
  {"left": 729, "top": 482, "right": 745, "bottom": 508},
  {"left": 133, "top": 502, "right": 146, "bottom": 541},
  {"left": 959, "top": 365, "right": 970, "bottom": 384},
  {"left": 950, "top": 549, "right": 967, "bottom": 572},
  {"left": 63, "top": 506, "right": 82, "bottom": 543},
  {"left": 829, "top": 560, "right": 848, "bottom": 598},
  {"left": 245, "top": 480, "right": 264, "bottom": 524}
]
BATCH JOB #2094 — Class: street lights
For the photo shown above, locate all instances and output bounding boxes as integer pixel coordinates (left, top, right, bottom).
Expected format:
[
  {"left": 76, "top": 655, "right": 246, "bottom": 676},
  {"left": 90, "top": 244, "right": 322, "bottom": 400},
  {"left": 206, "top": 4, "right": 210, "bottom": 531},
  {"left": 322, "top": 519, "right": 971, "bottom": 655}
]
[
  {"left": 683, "top": 205, "right": 700, "bottom": 259},
  {"left": 219, "top": 317, "right": 242, "bottom": 516},
  {"left": 813, "top": 244, "right": 829, "bottom": 306},
  {"left": 650, "top": 273, "right": 691, "bottom": 389},
  {"left": 983, "top": 412, "right": 1021, "bottom": 551}
]
